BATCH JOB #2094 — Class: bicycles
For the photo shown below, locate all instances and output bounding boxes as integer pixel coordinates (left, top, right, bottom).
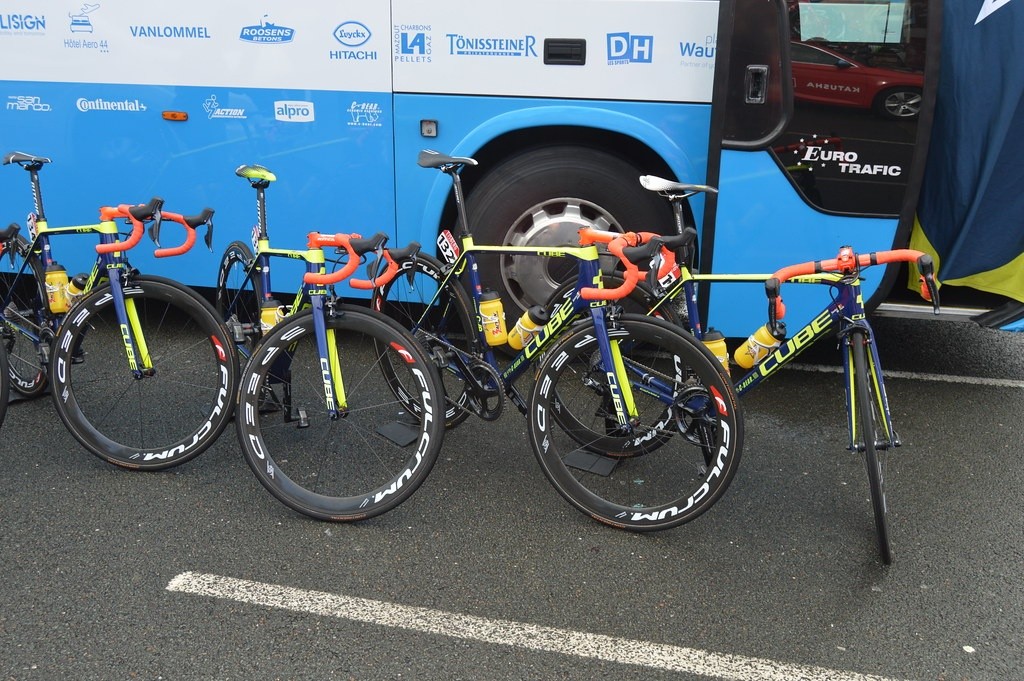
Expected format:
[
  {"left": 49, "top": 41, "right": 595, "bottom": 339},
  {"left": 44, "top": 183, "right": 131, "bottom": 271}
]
[
  {"left": 0, "top": 146, "right": 239, "bottom": 472},
  {"left": 364, "top": 145, "right": 745, "bottom": 533},
  {"left": 213, "top": 162, "right": 444, "bottom": 522},
  {"left": 536, "top": 171, "right": 943, "bottom": 567}
]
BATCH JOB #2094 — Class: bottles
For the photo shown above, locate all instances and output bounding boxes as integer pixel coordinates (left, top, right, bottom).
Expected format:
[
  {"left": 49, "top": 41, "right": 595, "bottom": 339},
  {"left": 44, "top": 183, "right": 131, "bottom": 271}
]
[
  {"left": 261, "top": 295, "right": 284, "bottom": 338},
  {"left": 45, "top": 260, "right": 69, "bottom": 314},
  {"left": 508, "top": 304, "right": 550, "bottom": 349},
  {"left": 734, "top": 320, "right": 787, "bottom": 369},
  {"left": 702, "top": 324, "right": 731, "bottom": 382},
  {"left": 64, "top": 271, "right": 89, "bottom": 314},
  {"left": 479, "top": 285, "right": 509, "bottom": 347}
]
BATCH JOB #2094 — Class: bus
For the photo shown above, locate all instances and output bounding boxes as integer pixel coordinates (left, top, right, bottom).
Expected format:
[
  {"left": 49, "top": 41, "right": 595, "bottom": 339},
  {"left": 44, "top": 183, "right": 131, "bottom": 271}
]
[{"left": 2, "top": 2, "right": 1022, "bottom": 416}]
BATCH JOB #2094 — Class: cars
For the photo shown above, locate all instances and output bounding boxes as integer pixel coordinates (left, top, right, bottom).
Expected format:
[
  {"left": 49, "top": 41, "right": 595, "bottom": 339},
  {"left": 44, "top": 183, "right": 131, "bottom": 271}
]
[{"left": 767, "top": 31, "right": 925, "bottom": 122}]
